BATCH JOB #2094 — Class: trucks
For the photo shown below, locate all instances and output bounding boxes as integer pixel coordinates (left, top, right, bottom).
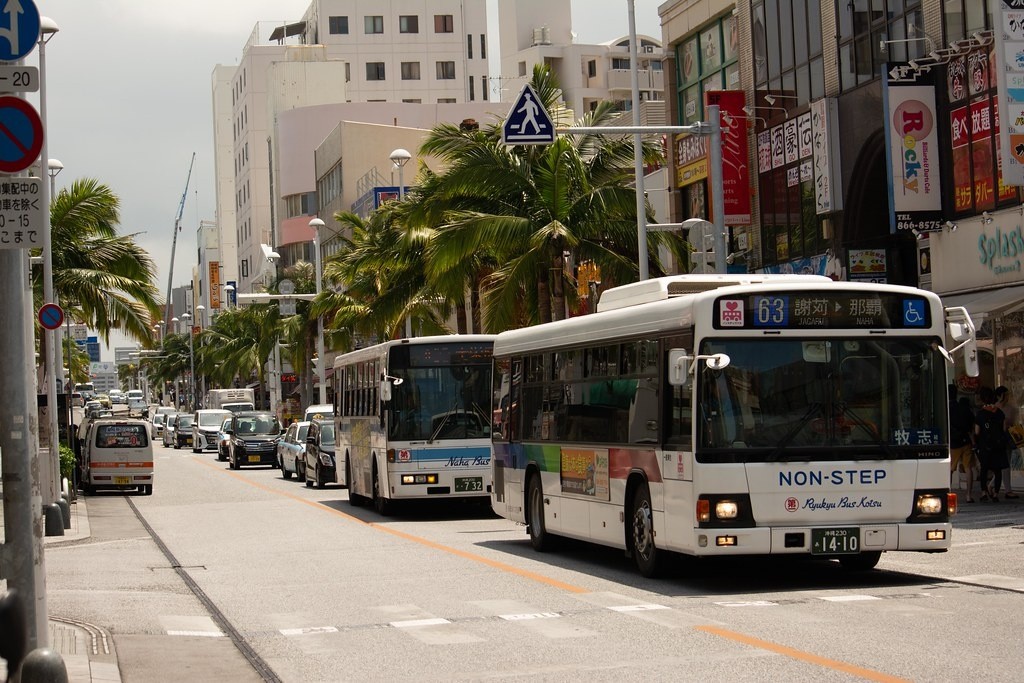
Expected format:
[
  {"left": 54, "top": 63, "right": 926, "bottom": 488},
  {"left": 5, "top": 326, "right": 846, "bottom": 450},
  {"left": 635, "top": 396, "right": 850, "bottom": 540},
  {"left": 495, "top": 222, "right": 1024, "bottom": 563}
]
[{"left": 205, "top": 388, "right": 255, "bottom": 418}]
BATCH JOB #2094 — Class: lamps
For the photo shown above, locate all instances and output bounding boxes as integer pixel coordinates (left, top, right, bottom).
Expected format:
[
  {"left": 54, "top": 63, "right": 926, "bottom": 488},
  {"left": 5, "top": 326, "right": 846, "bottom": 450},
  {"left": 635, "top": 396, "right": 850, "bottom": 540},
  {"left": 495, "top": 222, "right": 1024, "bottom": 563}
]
[
  {"left": 879, "top": 38, "right": 933, "bottom": 54},
  {"left": 975, "top": 29, "right": 995, "bottom": 45},
  {"left": 726, "top": 243, "right": 759, "bottom": 264},
  {"left": 982, "top": 207, "right": 1024, "bottom": 225},
  {"left": 888, "top": 49, "right": 951, "bottom": 81},
  {"left": 723, "top": 115, "right": 767, "bottom": 128},
  {"left": 928, "top": 45, "right": 981, "bottom": 63},
  {"left": 946, "top": 217, "right": 985, "bottom": 233},
  {"left": 911, "top": 225, "right": 950, "bottom": 240},
  {"left": 764, "top": 94, "right": 811, "bottom": 108},
  {"left": 742, "top": 106, "right": 789, "bottom": 119},
  {"left": 948, "top": 36, "right": 995, "bottom": 53},
  {"left": 908, "top": 23, "right": 936, "bottom": 52},
  {"left": 908, "top": 54, "right": 963, "bottom": 72}
]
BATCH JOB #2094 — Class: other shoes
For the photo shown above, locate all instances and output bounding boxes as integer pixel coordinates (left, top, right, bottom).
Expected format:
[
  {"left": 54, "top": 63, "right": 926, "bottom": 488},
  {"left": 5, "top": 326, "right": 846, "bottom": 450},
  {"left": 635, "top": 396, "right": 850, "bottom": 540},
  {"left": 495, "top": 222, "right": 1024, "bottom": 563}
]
[
  {"left": 992, "top": 496, "right": 999, "bottom": 503},
  {"left": 1004, "top": 491, "right": 1020, "bottom": 499},
  {"left": 966, "top": 497, "right": 974, "bottom": 503},
  {"left": 988, "top": 492, "right": 993, "bottom": 499},
  {"left": 980, "top": 494, "right": 989, "bottom": 502}
]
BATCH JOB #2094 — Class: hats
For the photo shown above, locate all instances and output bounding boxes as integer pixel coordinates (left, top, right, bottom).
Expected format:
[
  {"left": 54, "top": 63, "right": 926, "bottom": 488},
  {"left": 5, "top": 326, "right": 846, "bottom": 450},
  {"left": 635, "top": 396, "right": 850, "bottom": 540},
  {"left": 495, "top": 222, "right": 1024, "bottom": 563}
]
[{"left": 980, "top": 386, "right": 997, "bottom": 405}]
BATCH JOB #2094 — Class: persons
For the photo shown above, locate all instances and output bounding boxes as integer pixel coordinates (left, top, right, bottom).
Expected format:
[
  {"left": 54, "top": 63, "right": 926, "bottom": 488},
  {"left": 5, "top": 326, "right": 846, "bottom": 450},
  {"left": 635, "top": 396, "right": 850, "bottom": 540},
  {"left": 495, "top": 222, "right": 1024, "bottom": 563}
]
[
  {"left": 241, "top": 421, "right": 271, "bottom": 433},
  {"left": 400, "top": 393, "right": 430, "bottom": 423},
  {"left": 157, "top": 387, "right": 186, "bottom": 407},
  {"left": 949, "top": 383, "right": 1020, "bottom": 503}
]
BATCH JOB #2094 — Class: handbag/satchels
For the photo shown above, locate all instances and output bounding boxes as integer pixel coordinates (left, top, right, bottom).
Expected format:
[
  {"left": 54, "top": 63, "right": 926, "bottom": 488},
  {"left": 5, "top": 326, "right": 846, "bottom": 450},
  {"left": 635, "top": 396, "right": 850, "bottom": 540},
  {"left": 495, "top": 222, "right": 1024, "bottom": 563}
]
[{"left": 1004, "top": 429, "right": 1018, "bottom": 450}]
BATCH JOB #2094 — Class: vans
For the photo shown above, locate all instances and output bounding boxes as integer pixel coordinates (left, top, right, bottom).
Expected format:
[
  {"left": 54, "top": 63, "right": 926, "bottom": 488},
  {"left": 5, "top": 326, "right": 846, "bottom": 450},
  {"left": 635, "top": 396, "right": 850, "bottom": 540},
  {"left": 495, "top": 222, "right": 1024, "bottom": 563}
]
[{"left": 191, "top": 409, "right": 232, "bottom": 455}]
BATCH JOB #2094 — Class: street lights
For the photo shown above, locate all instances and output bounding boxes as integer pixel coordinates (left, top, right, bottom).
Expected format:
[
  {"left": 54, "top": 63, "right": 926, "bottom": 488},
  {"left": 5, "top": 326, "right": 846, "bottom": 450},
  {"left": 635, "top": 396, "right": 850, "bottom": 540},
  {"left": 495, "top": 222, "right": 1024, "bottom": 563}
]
[
  {"left": 390, "top": 150, "right": 412, "bottom": 335},
  {"left": 171, "top": 317, "right": 179, "bottom": 337},
  {"left": 223, "top": 286, "right": 235, "bottom": 313},
  {"left": 151, "top": 319, "right": 164, "bottom": 349},
  {"left": 180, "top": 312, "right": 191, "bottom": 333},
  {"left": 201, "top": 303, "right": 207, "bottom": 411},
  {"left": 308, "top": 217, "right": 325, "bottom": 403}
]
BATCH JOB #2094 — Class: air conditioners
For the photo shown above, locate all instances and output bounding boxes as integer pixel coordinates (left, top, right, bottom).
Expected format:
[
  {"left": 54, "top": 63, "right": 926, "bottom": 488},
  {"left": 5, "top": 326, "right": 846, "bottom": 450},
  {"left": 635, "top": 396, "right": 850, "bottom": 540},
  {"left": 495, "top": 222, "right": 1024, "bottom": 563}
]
[{"left": 644, "top": 45, "right": 658, "bottom": 54}]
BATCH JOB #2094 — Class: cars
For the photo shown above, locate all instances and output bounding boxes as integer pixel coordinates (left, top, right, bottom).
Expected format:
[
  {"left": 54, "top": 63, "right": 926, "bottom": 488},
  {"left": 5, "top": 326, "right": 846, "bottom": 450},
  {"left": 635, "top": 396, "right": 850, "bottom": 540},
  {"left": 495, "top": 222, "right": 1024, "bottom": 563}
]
[
  {"left": 303, "top": 403, "right": 334, "bottom": 421},
  {"left": 225, "top": 410, "right": 284, "bottom": 470},
  {"left": 150, "top": 414, "right": 165, "bottom": 441},
  {"left": 68, "top": 380, "right": 154, "bottom": 497},
  {"left": 275, "top": 421, "right": 311, "bottom": 483},
  {"left": 215, "top": 418, "right": 254, "bottom": 461},
  {"left": 304, "top": 417, "right": 336, "bottom": 492},
  {"left": 171, "top": 413, "right": 199, "bottom": 450},
  {"left": 154, "top": 406, "right": 178, "bottom": 416},
  {"left": 160, "top": 416, "right": 178, "bottom": 448}
]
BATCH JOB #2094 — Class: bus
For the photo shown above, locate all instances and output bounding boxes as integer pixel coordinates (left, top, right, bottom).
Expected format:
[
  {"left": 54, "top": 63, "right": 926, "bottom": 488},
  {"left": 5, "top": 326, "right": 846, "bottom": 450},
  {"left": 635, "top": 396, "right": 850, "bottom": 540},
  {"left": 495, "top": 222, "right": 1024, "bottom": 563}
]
[
  {"left": 486, "top": 274, "right": 981, "bottom": 581},
  {"left": 332, "top": 333, "right": 493, "bottom": 518}
]
